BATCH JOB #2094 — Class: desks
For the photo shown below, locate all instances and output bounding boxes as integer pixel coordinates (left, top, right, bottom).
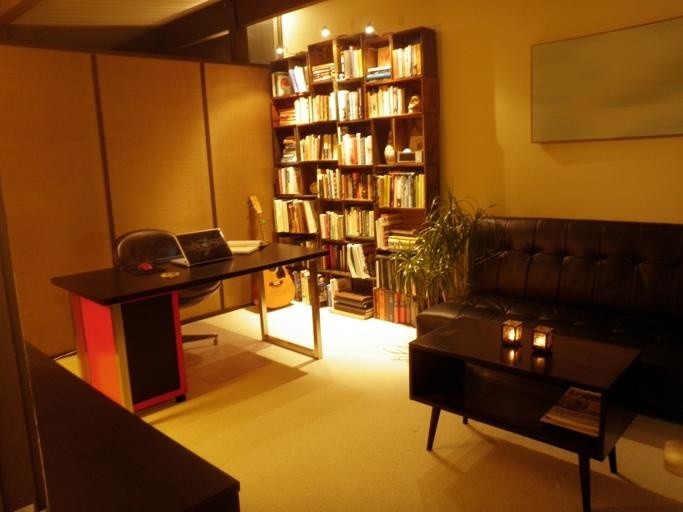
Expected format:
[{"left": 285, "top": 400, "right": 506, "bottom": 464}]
[{"left": 50, "top": 242, "right": 331, "bottom": 414}]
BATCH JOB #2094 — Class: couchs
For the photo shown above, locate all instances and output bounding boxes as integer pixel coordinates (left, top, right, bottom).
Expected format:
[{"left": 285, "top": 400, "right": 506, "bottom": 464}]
[{"left": 416, "top": 216, "right": 683, "bottom": 423}]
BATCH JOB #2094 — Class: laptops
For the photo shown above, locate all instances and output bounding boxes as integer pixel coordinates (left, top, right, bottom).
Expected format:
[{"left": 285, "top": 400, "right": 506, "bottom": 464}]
[{"left": 170, "top": 227, "right": 234, "bottom": 268}]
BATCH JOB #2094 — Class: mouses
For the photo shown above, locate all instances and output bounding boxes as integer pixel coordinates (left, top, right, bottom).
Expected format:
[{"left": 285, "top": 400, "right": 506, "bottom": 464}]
[{"left": 136, "top": 262, "right": 153, "bottom": 271}]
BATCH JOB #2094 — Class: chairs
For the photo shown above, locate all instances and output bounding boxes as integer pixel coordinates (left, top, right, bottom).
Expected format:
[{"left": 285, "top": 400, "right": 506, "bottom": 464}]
[{"left": 112, "top": 229, "right": 221, "bottom": 345}]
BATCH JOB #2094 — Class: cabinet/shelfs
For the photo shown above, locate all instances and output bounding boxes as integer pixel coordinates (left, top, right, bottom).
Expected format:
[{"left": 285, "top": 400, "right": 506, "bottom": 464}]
[{"left": 270, "top": 25, "right": 438, "bottom": 318}]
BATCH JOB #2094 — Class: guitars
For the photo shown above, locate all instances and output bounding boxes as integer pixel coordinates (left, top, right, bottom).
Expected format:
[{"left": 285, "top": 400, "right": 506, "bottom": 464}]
[{"left": 248, "top": 193, "right": 295, "bottom": 309}]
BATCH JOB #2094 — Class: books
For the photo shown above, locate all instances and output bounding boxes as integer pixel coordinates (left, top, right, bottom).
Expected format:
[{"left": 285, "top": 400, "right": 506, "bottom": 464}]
[
  {"left": 540, "top": 414, "right": 599, "bottom": 437},
  {"left": 226, "top": 237, "right": 270, "bottom": 255},
  {"left": 281, "top": 133, "right": 373, "bottom": 165},
  {"left": 544, "top": 386, "right": 600, "bottom": 433},
  {"left": 319, "top": 206, "right": 374, "bottom": 241},
  {"left": 371, "top": 171, "right": 426, "bottom": 209},
  {"left": 279, "top": 237, "right": 375, "bottom": 279},
  {"left": 273, "top": 44, "right": 421, "bottom": 98},
  {"left": 376, "top": 254, "right": 418, "bottom": 295},
  {"left": 318, "top": 168, "right": 372, "bottom": 200},
  {"left": 292, "top": 269, "right": 352, "bottom": 308},
  {"left": 375, "top": 213, "right": 424, "bottom": 250},
  {"left": 273, "top": 199, "right": 317, "bottom": 233},
  {"left": 274, "top": 165, "right": 301, "bottom": 194},
  {"left": 334, "top": 286, "right": 374, "bottom": 320}
]
